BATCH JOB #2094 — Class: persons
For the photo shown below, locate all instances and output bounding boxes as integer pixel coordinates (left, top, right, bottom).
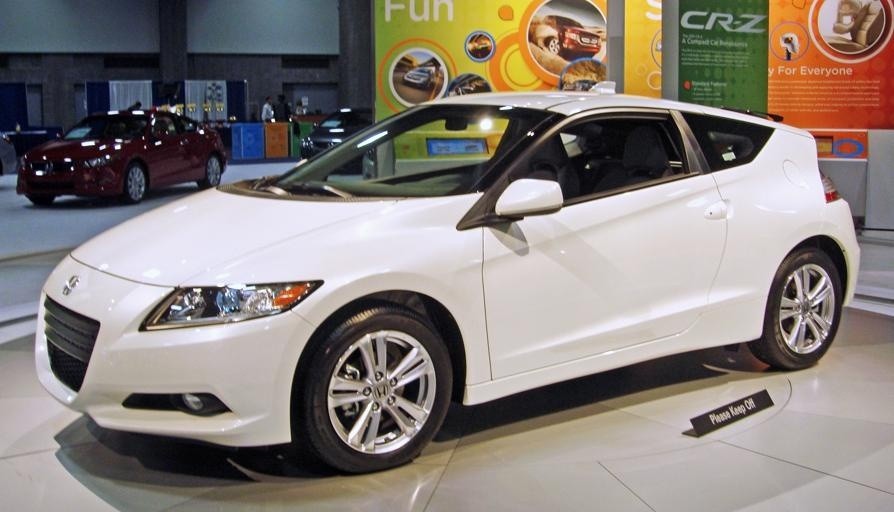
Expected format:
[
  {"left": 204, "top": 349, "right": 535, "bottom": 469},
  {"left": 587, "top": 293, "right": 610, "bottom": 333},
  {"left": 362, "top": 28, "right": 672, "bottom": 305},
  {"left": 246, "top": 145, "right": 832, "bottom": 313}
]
[
  {"left": 273, "top": 94, "right": 291, "bottom": 121},
  {"left": 294, "top": 101, "right": 306, "bottom": 115},
  {"left": 261, "top": 96, "right": 273, "bottom": 123},
  {"left": 128, "top": 101, "right": 141, "bottom": 111}
]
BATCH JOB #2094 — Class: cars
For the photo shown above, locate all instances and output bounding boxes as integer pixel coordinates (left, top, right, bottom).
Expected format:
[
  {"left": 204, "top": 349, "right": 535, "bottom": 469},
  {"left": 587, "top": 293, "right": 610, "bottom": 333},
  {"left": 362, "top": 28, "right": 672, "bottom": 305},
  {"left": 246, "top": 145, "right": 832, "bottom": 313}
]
[
  {"left": 535, "top": 15, "right": 602, "bottom": 56},
  {"left": 33, "top": 89, "right": 862, "bottom": 477},
  {"left": 300, "top": 107, "right": 372, "bottom": 175},
  {"left": 0, "top": 132, "right": 18, "bottom": 176},
  {"left": 402, "top": 67, "right": 436, "bottom": 89},
  {"left": 16, "top": 108, "right": 229, "bottom": 206}
]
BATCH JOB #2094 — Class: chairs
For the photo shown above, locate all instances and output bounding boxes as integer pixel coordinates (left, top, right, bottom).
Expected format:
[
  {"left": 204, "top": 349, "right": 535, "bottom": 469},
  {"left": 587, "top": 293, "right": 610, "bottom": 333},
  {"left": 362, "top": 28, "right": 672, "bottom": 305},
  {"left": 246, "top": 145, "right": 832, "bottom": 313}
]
[{"left": 529, "top": 124, "right": 676, "bottom": 199}]
